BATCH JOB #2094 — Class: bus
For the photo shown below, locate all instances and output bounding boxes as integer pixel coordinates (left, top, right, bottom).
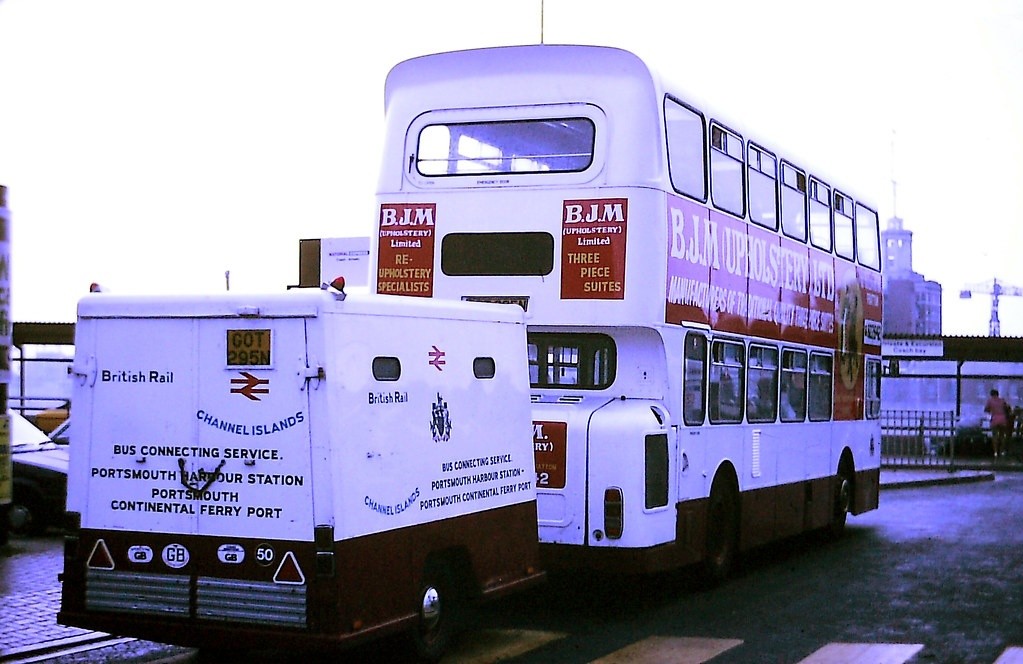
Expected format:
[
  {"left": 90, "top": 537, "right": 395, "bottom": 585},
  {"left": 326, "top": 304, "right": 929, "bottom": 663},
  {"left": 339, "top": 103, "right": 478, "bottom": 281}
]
[{"left": 365, "top": 43, "right": 883, "bottom": 592}]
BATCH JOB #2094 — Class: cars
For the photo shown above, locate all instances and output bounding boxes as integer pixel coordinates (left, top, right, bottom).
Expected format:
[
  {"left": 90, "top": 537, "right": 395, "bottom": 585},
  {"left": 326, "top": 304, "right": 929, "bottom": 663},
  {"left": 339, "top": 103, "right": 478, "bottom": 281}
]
[{"left": 7, "top": 408, "right": 79, "bottom": 543}]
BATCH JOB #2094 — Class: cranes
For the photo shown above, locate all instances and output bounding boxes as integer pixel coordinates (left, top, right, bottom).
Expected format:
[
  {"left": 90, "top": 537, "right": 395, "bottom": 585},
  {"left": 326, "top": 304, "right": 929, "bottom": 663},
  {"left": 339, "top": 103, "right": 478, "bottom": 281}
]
[{"left": 958, "top": 276, "right": 1023, "bottom": 337}]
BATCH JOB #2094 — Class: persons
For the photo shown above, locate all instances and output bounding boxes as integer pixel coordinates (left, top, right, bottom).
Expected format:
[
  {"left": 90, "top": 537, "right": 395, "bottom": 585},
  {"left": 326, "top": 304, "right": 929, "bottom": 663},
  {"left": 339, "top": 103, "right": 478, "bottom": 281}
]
[
  {"left": 956, "top": 416, "right": 985, "bottom": 458},
  {"left": 984, "top": 389, "right": 1012, "bottom": 458},
  {"left": 682, "top": 377, "right": 830, "bottom": 423}
]
